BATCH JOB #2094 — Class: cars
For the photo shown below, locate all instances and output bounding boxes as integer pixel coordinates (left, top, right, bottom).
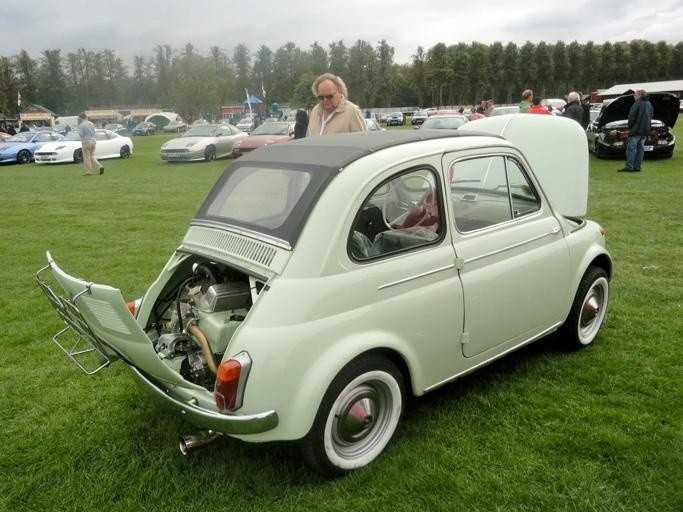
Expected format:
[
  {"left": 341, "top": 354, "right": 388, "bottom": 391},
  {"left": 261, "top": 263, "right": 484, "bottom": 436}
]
[
  {"left": 230, "top": 119, "right": 298, "bottom": 158},
  {"left": 0, "top": 130, "right": 66, "bottom": 166},
  {"left": 32, "top": 127, "right": 134, "bottom": 165},
  {"left": 28, "top": 108, "right": 618, "bottom": 488},
  {"left": 540, "top": 98, "right": 568, "bottom": 114},
  {"left": 581, "top": 91, "right": 682, "bottom": 162}
]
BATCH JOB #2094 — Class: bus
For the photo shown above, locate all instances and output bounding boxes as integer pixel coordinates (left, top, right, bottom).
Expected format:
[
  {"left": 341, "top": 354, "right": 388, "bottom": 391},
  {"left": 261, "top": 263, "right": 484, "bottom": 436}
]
[{"left": 157, "top": 121, "right": 246, "bottom": 164}]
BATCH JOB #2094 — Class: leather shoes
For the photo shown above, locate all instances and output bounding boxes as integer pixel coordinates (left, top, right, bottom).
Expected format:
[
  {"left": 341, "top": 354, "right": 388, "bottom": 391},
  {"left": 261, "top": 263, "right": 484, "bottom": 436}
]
[{"left": 617, "top": 169, "right": 631, "bottom": 172}]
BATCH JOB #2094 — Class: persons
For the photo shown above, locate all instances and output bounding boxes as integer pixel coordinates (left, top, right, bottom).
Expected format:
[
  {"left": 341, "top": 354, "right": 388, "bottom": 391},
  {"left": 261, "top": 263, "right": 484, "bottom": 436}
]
[
  {"left": 617, "top": 89, "right": 654, "bottom": 172},
  {"left": 79, "top": 112, "right": 104, "bottom": 176},
  {"left": 459, "top": 89, "right": 590, "bottom": 129},
  {"left": 305, "top": 73, "right": 367, "bottom": 138},
  {"left": 294, "top": 106, "right": 309, "bottom": 139},
  {"left": 20, "top": 123, "right": 29, "bottom": 132},
  {"left": 7, "top": 123, "right": 15, "bottom": 135}
]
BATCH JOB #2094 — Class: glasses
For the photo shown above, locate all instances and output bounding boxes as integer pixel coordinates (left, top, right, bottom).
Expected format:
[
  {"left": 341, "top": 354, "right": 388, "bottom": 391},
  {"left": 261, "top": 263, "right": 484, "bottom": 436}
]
[{"left": 318, "top": 88, "right": 337, "bottom": 100}]
